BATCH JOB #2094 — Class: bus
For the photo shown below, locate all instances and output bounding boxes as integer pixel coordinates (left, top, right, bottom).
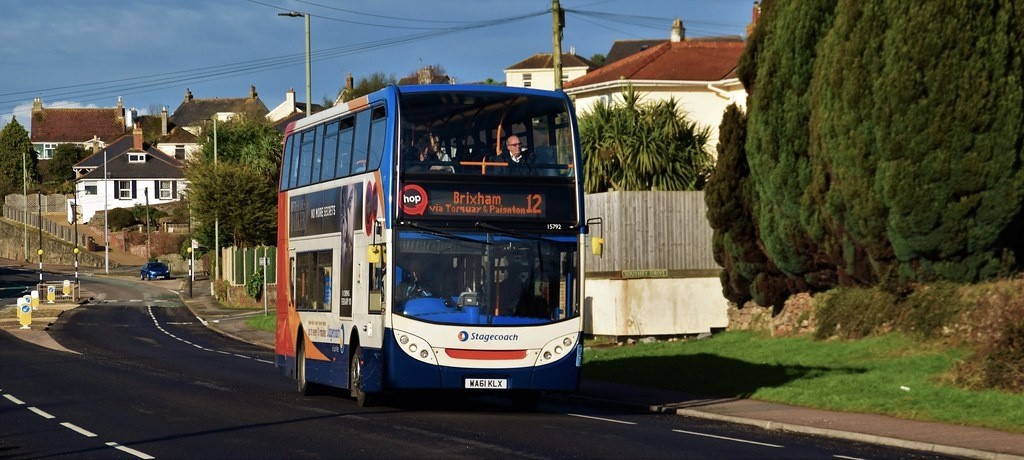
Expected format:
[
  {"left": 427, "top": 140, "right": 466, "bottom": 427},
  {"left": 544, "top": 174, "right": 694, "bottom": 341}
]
[{"left": 271, "top": 81, "right": 603, "bottom": 407}]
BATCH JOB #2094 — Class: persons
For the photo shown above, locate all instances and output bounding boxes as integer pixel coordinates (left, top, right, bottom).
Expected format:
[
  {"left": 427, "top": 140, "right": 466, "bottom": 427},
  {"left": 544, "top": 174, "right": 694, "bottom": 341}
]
[
  {"left": 489, "top": 135, "right": 541, "bottom": 177},
  {"left": 497, "top": 261, "right": 523, "bottom": 316},
  {"left": 395, "top": 259, "right": 433, "bottom": 301},
  {"left": 419, "top": 131, "right": 456, "bottom": 173}
]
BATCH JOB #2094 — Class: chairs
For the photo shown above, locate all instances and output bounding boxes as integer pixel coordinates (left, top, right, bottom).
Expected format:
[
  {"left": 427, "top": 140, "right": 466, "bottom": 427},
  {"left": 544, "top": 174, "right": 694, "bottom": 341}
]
[{"left": 311, "top": 145, "right": 559, "bottom": 182}]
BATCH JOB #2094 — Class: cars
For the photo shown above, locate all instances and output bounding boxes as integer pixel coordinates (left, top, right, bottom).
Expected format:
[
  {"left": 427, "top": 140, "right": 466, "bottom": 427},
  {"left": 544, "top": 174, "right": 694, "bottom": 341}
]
[{"left": 140, "top": 263, "right": 170, "bottom": 281}]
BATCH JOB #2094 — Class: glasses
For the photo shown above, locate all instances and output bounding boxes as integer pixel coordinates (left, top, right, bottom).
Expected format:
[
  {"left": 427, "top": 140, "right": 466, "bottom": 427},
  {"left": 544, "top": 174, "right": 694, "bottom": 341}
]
[
  {"left": 434, "top": 141, "right": 440, "bottom": 145},
  {"left": 506, "top": 142, "right": 524, "bottom": 148}
]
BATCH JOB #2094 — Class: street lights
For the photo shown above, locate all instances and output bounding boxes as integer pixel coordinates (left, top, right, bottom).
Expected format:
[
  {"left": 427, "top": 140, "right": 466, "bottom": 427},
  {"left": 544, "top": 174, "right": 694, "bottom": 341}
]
[
  {"left": 200, "top": 118, "right": 220, "bottom": 300},
  {"left": 277, "top": 11, "right": 311, "bottom": 117}
]
